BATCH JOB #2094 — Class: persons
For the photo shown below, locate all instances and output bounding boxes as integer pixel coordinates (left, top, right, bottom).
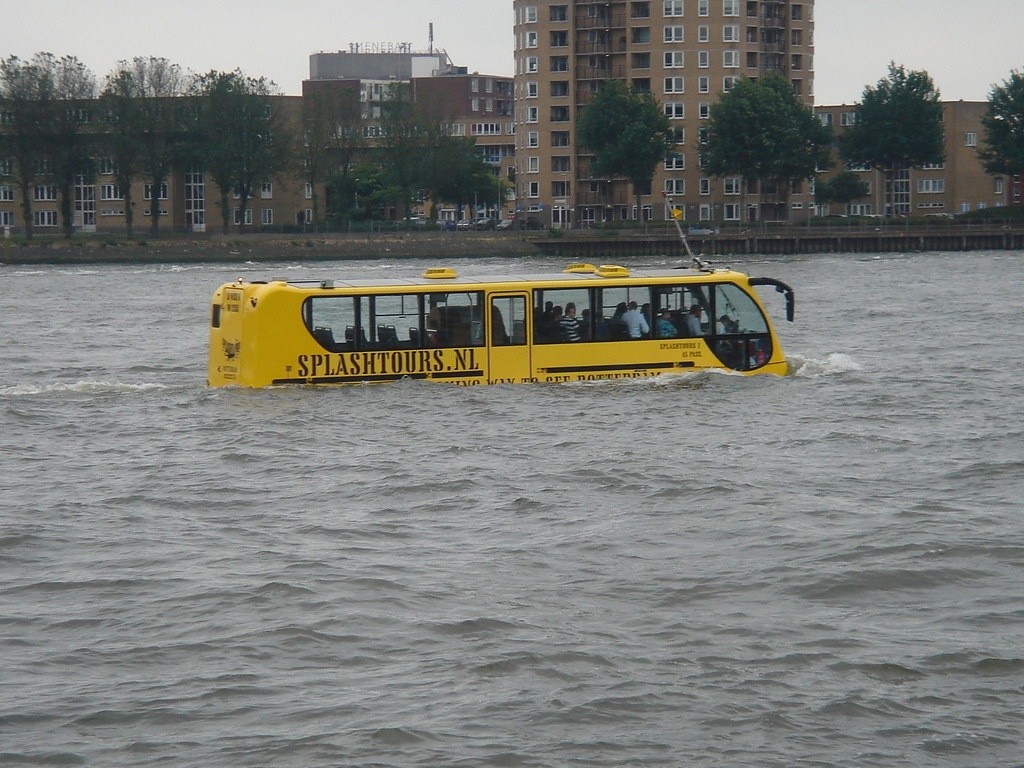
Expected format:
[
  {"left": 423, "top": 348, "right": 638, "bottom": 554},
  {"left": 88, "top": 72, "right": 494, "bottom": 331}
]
[
  {"left": 532, "top": 301, "right": 590, "bottom": 343},
  {"left": 658, "top": 308, "right": 678, "bottom": 338},
  {"left": 684, "top": 305, "right": 712, "bottom": 336},
  {"left": 611, "top": 301, "right": 651, "bottom": 339},
  {"left": 708, "top": 315, "right": 738, "bottom": 369}
]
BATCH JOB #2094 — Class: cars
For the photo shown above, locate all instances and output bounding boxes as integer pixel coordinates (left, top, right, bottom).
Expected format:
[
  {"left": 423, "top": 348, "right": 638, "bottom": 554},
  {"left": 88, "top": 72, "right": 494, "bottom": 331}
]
[
  {"left": 521, "top": 218, "right": 540, "bottom": 230},
  {"left": 472, "top": 217, "right": 502, "bottom": 230},
  {"left": 827, "top": 213, "right": 955, "bottom": 220},
  {"left": 440, "top": 219, "right": 456, "bottom": 231},
  {"left": 496, "top": 219, "right": 516, "bottom": 231},
  {"left": 457, "top": 220, "right": 469, "bottom": 230}
]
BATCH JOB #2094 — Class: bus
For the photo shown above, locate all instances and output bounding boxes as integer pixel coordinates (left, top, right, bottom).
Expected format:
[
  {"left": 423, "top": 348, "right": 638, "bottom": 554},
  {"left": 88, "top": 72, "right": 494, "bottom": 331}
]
[{"left": 205, "top": 258, "right": 795, "bottom": 388}]
